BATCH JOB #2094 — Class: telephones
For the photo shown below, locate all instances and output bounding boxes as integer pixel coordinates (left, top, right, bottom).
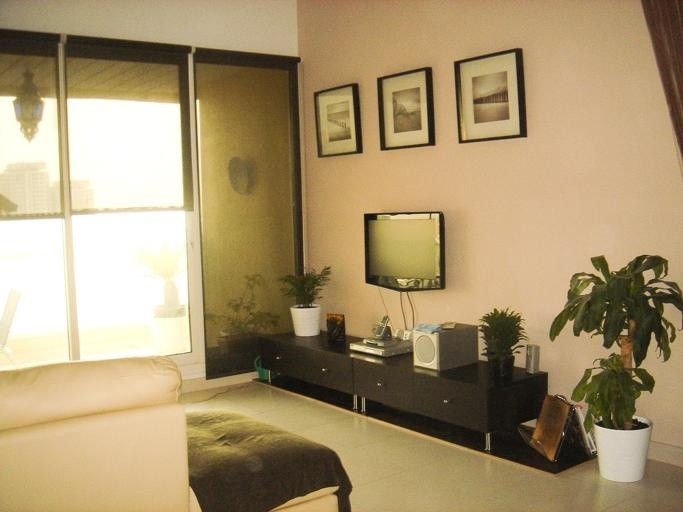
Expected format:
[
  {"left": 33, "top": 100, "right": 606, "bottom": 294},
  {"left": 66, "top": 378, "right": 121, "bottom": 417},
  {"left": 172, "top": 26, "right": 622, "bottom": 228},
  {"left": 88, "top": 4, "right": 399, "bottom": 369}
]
[{"left": 372, "top": 315, "right": 392, "bottom": 339}]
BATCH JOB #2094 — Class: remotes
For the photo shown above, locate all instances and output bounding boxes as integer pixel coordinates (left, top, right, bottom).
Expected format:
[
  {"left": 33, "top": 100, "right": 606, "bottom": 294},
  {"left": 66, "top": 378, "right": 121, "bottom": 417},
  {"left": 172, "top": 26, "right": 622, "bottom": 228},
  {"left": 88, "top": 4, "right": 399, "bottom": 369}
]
[{"left": 375, "top": 315, "right": 390, "bottom": 337}]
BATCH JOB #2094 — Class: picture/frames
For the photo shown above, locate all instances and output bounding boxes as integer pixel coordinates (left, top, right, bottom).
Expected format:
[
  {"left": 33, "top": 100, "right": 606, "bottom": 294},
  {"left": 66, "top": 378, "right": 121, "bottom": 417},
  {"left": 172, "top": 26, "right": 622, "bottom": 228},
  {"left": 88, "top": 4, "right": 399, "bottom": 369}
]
[
  {"left": 453, "top": 48, "right": 527, "bottom": 143},
  {"left": 377, "top": 67, "right": 435, "bottom": 150},
  {"left": 313, "top": 83, "right": 363, "bottom": 157}
]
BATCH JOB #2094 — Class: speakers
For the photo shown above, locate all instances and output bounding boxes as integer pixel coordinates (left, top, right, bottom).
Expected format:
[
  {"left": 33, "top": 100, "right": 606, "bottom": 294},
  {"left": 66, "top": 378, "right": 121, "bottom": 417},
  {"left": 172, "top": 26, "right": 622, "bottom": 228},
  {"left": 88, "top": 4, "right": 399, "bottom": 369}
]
[{"left": 414, "top": 322, "right": 478, "bottom": 372}]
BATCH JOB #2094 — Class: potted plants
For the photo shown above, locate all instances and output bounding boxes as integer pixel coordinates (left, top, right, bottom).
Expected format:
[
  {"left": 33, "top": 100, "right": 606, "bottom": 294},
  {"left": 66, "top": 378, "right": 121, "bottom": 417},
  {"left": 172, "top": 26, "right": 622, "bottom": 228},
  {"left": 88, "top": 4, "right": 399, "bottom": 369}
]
[
  {"left": 277, "top": 266, "right": 332, "bottom": 337},
  {"left": 477, "top": 307, "right": 529, "bottom": 369},
  {"left": 550, "top": 254, "right": 682, "bottom": 484},
  {"left": 204, "top": 272, "right": 281, "bottom": 373}
]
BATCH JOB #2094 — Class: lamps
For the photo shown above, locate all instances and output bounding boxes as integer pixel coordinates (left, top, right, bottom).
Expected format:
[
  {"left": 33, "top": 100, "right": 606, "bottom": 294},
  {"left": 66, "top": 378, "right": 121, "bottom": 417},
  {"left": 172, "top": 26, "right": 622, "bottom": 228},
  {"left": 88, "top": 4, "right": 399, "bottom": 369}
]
[{"left": 13, "top": 66, "right": 45, "bottom": 142}]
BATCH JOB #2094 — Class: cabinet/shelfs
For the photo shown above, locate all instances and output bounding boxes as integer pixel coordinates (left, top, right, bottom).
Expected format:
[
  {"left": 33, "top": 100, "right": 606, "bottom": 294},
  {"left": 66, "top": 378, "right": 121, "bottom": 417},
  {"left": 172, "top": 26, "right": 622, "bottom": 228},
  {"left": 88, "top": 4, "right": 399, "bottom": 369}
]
[{"left": 260, "top": 331, "right": 548, "bottom": 451}]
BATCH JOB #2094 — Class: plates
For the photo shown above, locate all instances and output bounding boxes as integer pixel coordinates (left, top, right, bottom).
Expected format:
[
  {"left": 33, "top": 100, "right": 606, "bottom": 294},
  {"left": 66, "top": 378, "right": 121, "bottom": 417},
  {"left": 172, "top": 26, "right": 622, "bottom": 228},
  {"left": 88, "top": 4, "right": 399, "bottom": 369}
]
[{"left": 226, "top": 156, "right": 249, "bottom": 194}]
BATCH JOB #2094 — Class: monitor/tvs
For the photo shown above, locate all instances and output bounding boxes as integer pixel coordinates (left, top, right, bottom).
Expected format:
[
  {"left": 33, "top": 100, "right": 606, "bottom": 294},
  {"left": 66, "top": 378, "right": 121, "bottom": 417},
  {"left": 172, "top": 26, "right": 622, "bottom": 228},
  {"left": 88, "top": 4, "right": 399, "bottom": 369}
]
[{"left": 364, "top": 211, "right": 445, "bottom": 291}]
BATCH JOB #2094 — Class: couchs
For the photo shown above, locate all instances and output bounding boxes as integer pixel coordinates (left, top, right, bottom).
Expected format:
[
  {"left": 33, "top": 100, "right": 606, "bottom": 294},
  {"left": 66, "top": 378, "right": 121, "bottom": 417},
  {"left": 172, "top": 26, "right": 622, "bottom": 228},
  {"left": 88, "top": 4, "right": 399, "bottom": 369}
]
[{"left": 0, "top": 354, "right": 354, "bottom": 512}]
